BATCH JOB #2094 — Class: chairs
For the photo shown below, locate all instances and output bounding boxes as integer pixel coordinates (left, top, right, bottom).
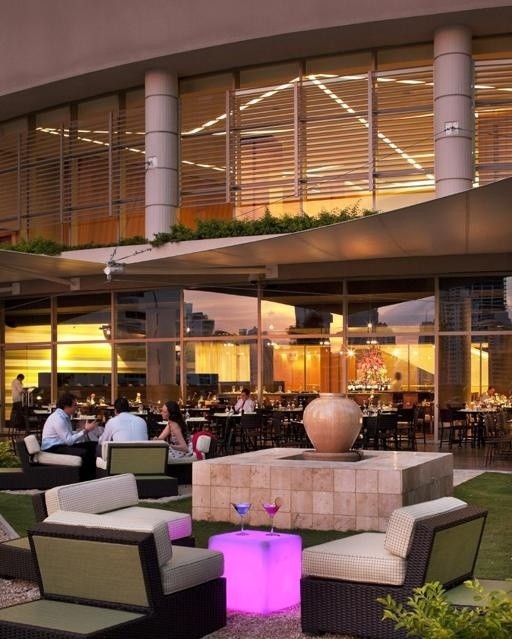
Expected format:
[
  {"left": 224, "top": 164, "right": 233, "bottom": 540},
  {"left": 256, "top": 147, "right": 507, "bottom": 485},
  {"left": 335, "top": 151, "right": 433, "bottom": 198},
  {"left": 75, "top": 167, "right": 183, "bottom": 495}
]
[
  {"left": 478, "top": 407, "right": 511, "bottom": 467},
  {"left": 438, "top": 402, "right": 468, "bottom": 450},
  {"left": 356, "top": 404, "right": 428, "bottom": 451},
  {"left": 232, "top": 409, "right": 262, "bottom": 454}
]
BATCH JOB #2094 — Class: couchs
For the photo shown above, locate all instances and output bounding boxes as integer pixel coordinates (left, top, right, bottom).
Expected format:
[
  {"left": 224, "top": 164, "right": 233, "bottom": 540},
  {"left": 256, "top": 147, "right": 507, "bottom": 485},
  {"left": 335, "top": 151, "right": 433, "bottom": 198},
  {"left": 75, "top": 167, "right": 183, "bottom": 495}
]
[
  {"left": 15, "top": 434, "right": 84, "bottom": 479},
  {"left": 26, "top": 509, "right": 228, "bottom": 636},
  {"left": 93, "top": 440, "right": 169, "bottom": 477},
  {"left": 298, "top": 495, "right": 489, "bottom": 637},
  {"left": 30, "top": 471, "right": 196, "bottom": 550},
  {"left": 166, "top": 434, "right": 212, "bottom": 484}
]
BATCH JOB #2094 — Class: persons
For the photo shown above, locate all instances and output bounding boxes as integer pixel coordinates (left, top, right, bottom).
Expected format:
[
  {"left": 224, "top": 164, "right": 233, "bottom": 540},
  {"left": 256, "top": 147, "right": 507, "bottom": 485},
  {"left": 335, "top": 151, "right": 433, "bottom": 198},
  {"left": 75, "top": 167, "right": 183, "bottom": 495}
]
[
  {"left": 221, "top": 389, "right": 255, "bottom": 450},
  {"left": 152, "top": 401, "right": 194, "bottom": 458},
  {"left": 40, "top": 393, "right": 99, "bottom": 482},
  {"left": 480, "top": 387, "right": 495, "bottom": 407},
  {"left": 391, "top": 371, "right": 401, "bottom": 391},
  {"left": 9, "top": 374, "right": 26, "bottom": 429},
  {"left": 98, "top": 397, "right": 148, "bottom": 445}
]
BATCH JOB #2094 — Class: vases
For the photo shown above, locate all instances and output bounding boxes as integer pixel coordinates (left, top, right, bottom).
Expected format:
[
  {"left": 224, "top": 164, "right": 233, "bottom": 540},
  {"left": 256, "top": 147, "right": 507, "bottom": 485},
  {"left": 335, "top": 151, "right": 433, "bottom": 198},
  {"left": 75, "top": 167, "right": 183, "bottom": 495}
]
[{"left": 303, "top": 390, "right": 362, "bottom": 453}]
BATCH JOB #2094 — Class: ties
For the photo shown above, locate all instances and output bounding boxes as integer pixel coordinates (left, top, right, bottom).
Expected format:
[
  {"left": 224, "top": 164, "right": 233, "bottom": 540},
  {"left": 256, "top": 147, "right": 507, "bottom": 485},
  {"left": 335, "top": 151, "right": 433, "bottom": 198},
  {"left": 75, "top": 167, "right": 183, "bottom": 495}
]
[{"left": 241, "top": 401, "right": 245, "bottom": 408}]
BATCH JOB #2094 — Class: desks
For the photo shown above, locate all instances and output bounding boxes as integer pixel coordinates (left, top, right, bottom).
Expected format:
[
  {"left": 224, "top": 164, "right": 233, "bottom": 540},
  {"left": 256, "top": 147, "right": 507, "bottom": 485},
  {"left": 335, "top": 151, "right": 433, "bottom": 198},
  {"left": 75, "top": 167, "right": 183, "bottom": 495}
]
[
  {"left": 213, "top": 412, "right": 256, "bottom": 418},
  {"left": 157, "top": 417, "right": 208, "bottom": 425},
  {"left": 271, "top": 406, "right": 302, "bottom": 419}
]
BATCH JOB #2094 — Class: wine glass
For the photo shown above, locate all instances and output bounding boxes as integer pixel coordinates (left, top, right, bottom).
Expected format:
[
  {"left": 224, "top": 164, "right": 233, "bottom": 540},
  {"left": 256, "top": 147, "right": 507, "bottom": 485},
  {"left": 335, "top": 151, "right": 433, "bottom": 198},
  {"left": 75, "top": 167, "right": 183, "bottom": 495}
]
[
  {"left": 229, "top": 405, "right": 235, "bottom": 415},
  {"left": 185, "top": 407, "right": 191, "bottom": 420},
  {"left": 224, "top": 405, "right": 229, "bottom": 414},
  {"left": 286, "top": 401, "right": 291, "bottom": 409},
  {"left": 232, "top": 502, "right": 253, "bottom": 536},
  {"left": 291, "top": 400, "right": 295, "bottom": 409},
  {"left": 261, "top": 501, "right": 282, "bottom": 537}
]
